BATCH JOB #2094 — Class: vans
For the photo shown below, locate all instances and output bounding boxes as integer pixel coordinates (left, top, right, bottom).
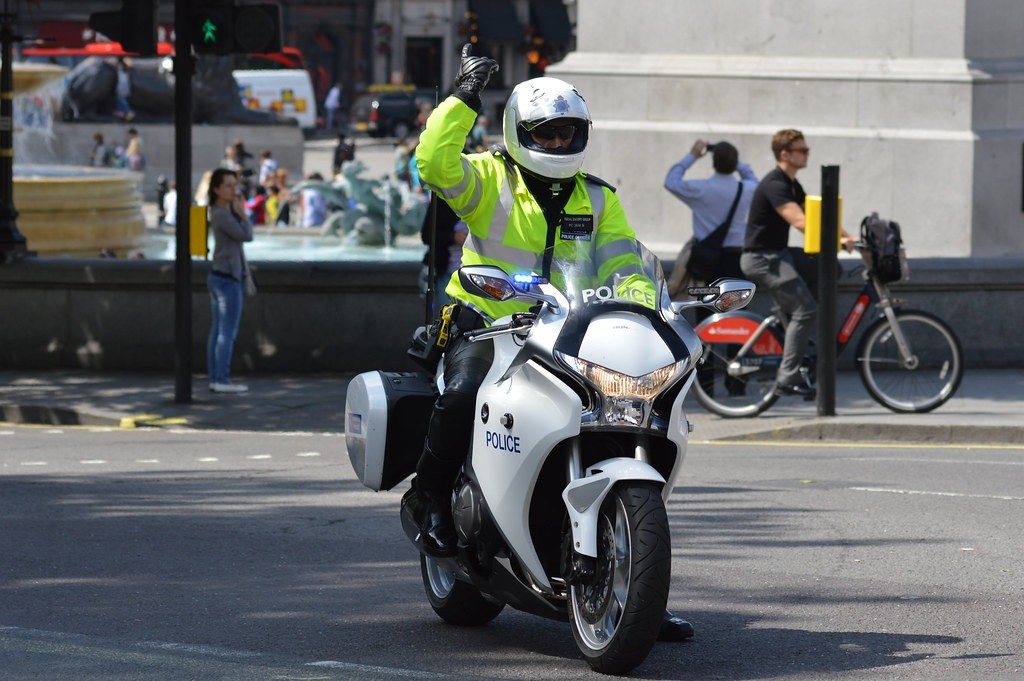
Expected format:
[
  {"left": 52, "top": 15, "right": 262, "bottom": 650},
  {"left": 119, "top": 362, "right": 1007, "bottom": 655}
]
[{"left": 232, "top": 69, "right": 316, "bottom": 140}]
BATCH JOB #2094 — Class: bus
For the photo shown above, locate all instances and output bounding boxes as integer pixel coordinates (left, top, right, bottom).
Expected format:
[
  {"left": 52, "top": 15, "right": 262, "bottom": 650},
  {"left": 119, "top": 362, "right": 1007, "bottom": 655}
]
[{"left": 14, "top": 43, "right": 329, "bottom": 126}]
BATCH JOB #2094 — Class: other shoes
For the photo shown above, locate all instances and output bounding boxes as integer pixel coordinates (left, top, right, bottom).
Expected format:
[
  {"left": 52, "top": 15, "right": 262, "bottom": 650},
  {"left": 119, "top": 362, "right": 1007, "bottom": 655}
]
[
  {"left": 775, "top": 382, "right": 819, "bottom": 398},
  {"left": 209, "top": 382, "right": 249, "bottom": 393}
]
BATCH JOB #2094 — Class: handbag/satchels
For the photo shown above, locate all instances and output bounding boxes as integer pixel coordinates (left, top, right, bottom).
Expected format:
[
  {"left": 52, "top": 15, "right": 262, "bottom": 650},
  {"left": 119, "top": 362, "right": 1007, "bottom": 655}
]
[
  {"left": 686, "top": 224, "right": 726, "bottom": 280},
  {"left": 243, "top": 274, "right": 257, "bottom": 297}
]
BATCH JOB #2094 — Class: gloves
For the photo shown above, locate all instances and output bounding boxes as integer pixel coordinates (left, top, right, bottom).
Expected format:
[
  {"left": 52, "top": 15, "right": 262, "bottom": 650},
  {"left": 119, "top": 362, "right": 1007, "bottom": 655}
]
[{"left": 455, "top": 42, "right": 501, "bottom": 94}]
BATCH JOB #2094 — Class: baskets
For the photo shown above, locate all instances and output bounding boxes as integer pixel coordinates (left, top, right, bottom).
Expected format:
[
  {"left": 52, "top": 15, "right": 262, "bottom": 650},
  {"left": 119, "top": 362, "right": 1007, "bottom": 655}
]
[{"left": 854, "top": 240, "right": 910, "bottom": 282}]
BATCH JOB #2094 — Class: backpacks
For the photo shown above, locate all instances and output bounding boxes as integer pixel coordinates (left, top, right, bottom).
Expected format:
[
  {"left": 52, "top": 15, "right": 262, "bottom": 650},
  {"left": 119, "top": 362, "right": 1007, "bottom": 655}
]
[{"left": 860, "top": 212, "right": 904, "bottom": 281}]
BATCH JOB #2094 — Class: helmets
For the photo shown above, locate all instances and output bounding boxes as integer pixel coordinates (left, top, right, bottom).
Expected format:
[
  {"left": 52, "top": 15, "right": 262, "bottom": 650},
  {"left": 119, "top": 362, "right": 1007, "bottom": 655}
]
[{"left": 502, "top": 77, "right": 592, "bottom": 182}]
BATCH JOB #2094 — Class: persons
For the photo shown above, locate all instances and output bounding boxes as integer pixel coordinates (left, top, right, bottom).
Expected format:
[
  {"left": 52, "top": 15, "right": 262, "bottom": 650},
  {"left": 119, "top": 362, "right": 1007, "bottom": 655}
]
[
  {"left": 90, "top": 127, "right": 147, "bottom": 173},
  {"left": 113, "top": 61, "right": 136, "bottom": 123},
  {"left": 740, "top": 127, "right": 855, "bottom": 401},
  {"left": 157, "top": 83, "right": 494, "bottom": 235},
  {"left": 400, "top": 44, "right": 696, "bottom": 643},
  {"left": 664, "top": 140, "right": 760, "bottom": 398},
  {"left": 95, "top": 249, "right": 145, "bottom": 259},
  {"left": 204, "top": 170, "right": 253, "bottom": 393}
]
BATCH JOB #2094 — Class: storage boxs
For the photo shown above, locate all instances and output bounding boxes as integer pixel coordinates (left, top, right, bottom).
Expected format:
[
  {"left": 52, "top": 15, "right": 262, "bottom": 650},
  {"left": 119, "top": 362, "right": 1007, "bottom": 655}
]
[{"left": 344, "top": 370, "right": 436, "bottom": 492}]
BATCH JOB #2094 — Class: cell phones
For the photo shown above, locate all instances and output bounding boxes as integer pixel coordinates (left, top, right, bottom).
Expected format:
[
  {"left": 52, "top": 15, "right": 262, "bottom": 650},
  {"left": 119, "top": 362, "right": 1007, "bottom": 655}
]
[
  {"left": 706, "top": 144, "right": 716, "bottom": 152},
  {"left": 243, "top": 169, "right": 255, "bottom": 176}
]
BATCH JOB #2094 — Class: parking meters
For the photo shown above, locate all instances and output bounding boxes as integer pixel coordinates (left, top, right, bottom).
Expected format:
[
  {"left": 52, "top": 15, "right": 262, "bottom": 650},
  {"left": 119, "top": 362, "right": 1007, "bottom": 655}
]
[{"left": 805, "top": 195, "right": 842, "bottom": 415}]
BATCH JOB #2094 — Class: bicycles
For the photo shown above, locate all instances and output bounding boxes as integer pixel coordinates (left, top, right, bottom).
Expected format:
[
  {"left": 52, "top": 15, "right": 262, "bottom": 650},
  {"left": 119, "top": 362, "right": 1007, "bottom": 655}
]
[{"left": 691, "top": 239, "right": 963, "bottom": 419}]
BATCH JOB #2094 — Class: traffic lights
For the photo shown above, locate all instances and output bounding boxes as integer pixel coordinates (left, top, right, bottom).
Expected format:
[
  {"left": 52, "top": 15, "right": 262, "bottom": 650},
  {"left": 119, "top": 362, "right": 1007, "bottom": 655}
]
[{"left": 190, "top": 10, "right": 234, "bottom": 57}]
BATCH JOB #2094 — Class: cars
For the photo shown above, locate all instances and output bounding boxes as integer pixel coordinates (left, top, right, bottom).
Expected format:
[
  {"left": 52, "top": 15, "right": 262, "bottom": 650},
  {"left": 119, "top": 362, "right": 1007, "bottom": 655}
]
[{"left": 348, "top": 93, "right": 419, "bottom": 138}]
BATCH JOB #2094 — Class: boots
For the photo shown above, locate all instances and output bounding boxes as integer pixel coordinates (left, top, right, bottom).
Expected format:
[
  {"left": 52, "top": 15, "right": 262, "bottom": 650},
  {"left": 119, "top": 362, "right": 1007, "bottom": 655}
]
[
  {"left": 615, "top": 606, "right": 695, "bottom": 640},
  {"left": 410, "top": 393, "right": 472, "bottom": 552}
]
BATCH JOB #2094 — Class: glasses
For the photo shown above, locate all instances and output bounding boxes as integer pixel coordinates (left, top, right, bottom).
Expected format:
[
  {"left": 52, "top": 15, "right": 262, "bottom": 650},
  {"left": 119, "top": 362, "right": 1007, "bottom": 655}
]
[
  {"left": 790, "top": 146, "right": 809, "bottom": 154},
  {"left": 534, "top": 124, "right": 576, "bottom": 141}
]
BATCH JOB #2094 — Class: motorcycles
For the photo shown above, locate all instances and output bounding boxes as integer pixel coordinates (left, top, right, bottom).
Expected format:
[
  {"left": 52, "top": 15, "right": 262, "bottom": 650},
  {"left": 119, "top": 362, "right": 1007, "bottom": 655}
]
[{"left": 343, "top": 266, "right": 756, "bottom": 674}]
